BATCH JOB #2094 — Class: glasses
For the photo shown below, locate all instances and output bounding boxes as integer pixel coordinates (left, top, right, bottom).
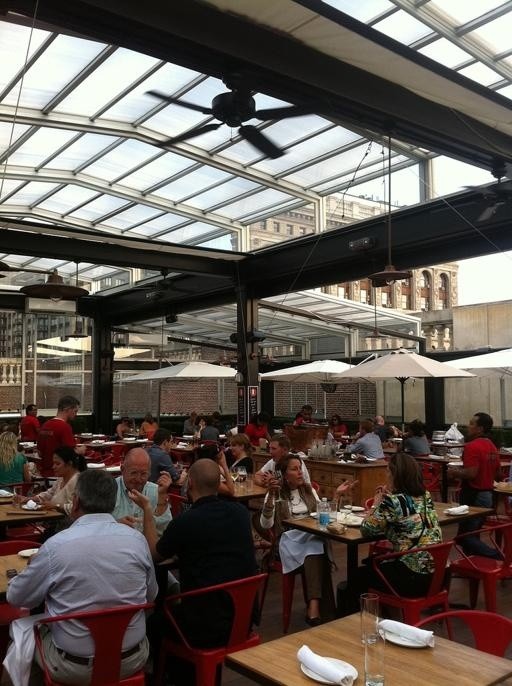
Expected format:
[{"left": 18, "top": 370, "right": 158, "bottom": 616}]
[{"left": 123, "top": 465, "right": 152, "bottom": 481}]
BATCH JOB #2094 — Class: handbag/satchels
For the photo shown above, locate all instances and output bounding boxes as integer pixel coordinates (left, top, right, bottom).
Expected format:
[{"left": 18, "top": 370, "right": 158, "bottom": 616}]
[{"left": 360, "top": 554, "right": 384, "bottom": 567}]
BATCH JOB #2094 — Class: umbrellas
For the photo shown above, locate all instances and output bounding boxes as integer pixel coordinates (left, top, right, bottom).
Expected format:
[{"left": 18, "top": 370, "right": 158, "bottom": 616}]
[
  {"left": 329, "top": 350, "right": 474, "bottom": 453},
  {"left": 442, "top": 347, "right": 512, "bottom": 378},
  {"left": 256, "top": 358, "right": 375, "bottom": 387},
  {"left": 120, "top": 358, "right": 242, "bottom": 383}
]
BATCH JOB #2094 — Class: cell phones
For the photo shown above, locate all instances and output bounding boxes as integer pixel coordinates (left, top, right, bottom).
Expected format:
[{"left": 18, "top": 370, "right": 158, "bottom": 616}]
[{"left": 7, "top": 569, "right": 17, "bottom": 578}]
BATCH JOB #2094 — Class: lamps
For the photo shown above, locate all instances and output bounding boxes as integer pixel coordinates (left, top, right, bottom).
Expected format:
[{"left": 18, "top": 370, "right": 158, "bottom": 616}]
[
  {"left": 370, "top": 121, "right": 414, "bottom": 288},
  {"left": 16, "top": 265, "right": 93, "bottom": 306}
]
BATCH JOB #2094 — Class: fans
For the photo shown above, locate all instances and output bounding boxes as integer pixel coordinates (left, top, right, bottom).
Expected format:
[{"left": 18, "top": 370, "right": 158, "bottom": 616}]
[
  {"left": 134, "top": 70, "right": 321, "bottom": 164},
  {"left": 126, "top": 266, "right": 196, "bottom": 295}
]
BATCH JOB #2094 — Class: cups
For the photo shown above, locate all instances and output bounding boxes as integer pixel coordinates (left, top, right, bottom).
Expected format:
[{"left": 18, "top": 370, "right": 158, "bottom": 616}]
[
  {"left": 133, "top": 510, "right": 145, "bottom": 534},
  {"left": 230, "top": 466, "right": 255, "bottom": 494},
  {"left": 308, "top": 438, "right": 338, "bottom": 458},
  {"left": 13, "top": 486, "right": 22, "bottom": 507},
  {"left": 316, "top": 495, "right": 352, "bottom": 533},
  {"left": 177, "top": 461, "right": 183, "bottom": 470},
  {"left": 359, "top": 592, "right": 380, "bottom": 643},
  {"left": 364, "top": 628, "right": 386, "bottom": 685}
]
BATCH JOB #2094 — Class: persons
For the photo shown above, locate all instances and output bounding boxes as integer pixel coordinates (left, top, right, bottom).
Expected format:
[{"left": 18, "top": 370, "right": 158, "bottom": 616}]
[
  {"left": 0, "top": 394, "right": 256, "bottom": 601},
  {"left": 443, "top": 412, "right": 503, "bottom": 579},
  {"left": 144, "top": 457, "right": 265, "bottom": 684},
  {"left": 0, "top": 468, "right": 159, "bottom": 686},
  {"left": 244, "top": 404, "right": 443, "bottom": 627}
]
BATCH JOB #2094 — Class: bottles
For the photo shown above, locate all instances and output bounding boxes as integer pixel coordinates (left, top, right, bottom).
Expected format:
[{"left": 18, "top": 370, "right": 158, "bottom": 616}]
[{"left": 346, "top": 442, "right": 351, "bottom": 459}]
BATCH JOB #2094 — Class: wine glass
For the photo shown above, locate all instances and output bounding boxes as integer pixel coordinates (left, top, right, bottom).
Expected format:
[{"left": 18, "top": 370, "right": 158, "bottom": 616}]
[{"left": 273, "top": 470, "right": 288, "bottom": 502}]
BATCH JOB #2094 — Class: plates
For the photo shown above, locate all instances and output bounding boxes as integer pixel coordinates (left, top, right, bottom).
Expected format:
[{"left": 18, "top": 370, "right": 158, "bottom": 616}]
[
  {"left": 300, "top": 656, "right": 358, "bottom": 683},
  {"left": 310, "top": 505, "right": 364, "bottom": 525},
  {"left": 444, "top": 509, "right": 470, "bottom": 515},
  {"left": 17, "top": 548, "right": 38, "bottom": 556},
  {"left": 433, "top": 441, "right": 446, "bottom": 444},
  {"left": 381, "top": 627, "right": 425, "bottom": 648},
  {"left": 448, "top": 461, "right": 464, "bottom": 466}
]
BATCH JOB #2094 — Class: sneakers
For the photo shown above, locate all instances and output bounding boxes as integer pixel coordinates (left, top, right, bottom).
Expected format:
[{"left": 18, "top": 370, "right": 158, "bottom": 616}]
[{"left": 304, "top": 613, "right": 323, "bottom": 628}]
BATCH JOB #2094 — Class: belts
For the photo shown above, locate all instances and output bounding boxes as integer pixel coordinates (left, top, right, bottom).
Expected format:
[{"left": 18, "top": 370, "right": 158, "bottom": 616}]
[{"left": 56, "top": 642, "right": 140, "bottom": 667}]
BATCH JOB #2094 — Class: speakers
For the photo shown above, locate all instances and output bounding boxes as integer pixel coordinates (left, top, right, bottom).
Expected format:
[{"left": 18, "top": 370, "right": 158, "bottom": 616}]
[
  {"left": 247, "top": 330, "right": 266, "bottom": 342},
  {"left": 166, "top": 315, "right": 178, "bottom": 323},
  {"left": 230, "top": 333, "right": 237, "bottom": 343},
  {"left": 102, "top": 350, "right": 114, "bottom": 358}
]
